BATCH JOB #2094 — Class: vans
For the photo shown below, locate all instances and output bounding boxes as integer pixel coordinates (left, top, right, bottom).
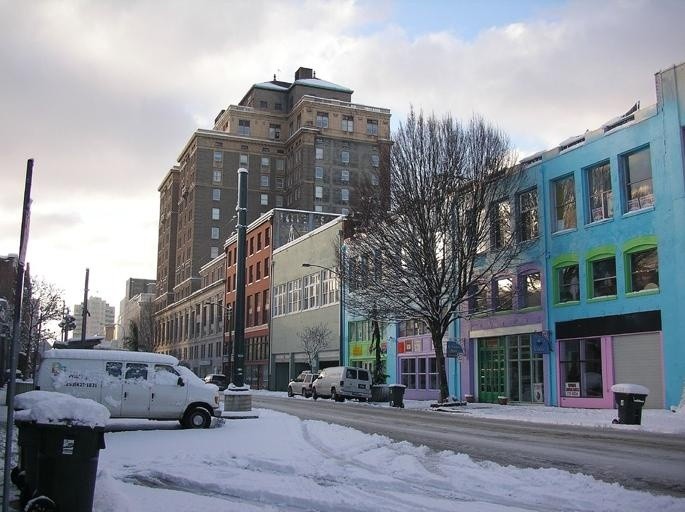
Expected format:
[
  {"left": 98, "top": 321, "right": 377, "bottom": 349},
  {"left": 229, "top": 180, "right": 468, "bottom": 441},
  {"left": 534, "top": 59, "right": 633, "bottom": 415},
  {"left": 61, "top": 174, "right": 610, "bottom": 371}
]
[
  {"left": 287, "top": 366, "right": 372, "bottom": 402},
  {"left": 35, "top": 349, "right": 219, "bottom": 428}
]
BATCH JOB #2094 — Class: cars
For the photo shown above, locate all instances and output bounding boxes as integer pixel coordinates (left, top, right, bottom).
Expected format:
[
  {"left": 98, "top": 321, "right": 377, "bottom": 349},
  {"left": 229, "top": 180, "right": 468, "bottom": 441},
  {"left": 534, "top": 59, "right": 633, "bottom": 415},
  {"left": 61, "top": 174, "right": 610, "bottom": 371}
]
[{"left": 203, "top": 374, "right": 229, "bottom": 391}]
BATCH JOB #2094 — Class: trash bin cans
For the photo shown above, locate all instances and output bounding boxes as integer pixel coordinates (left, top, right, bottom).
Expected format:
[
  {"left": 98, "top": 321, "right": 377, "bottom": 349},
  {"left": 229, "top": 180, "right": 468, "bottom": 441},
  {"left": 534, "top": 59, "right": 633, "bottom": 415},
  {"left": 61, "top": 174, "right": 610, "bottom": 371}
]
[
  {"left": 11, "top": 419, "right": 105, "bottom": 512},
  {"left": 388, "top": 386, "right": 407, "bottom": 408},
  {"left": 612, "top": 392, "right": 648, "bottom": 425}
]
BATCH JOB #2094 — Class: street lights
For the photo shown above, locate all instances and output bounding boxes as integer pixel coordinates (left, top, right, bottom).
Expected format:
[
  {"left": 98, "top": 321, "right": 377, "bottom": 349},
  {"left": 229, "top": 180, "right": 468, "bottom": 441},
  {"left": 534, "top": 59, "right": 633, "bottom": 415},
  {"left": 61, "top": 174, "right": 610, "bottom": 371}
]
[
  {"left": 205, "top": 300, "right": 233, "bottom": 388},
  {"left": 301, "top": 230, "right": 344, "bottom": 366}
]
